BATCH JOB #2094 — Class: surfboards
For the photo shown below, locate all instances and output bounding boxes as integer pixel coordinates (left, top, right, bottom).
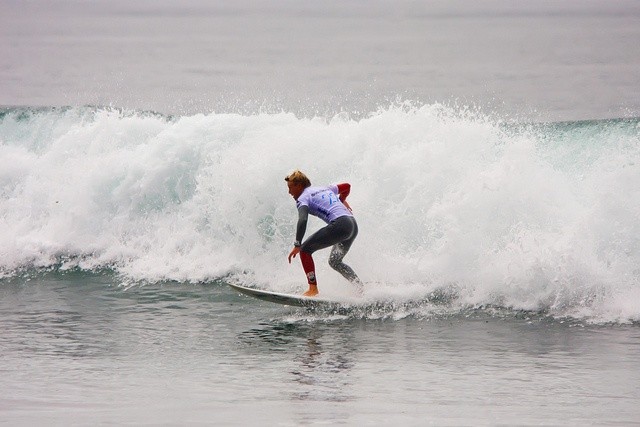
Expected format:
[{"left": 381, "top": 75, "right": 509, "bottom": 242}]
[{"left": 228, "top": 280, "right": 338, "bottom": 307}]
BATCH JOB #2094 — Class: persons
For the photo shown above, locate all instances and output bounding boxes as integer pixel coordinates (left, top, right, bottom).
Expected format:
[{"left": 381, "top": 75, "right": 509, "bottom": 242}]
[{"left": 284, "top": 171, "right": 366, "bottom": 297}]
